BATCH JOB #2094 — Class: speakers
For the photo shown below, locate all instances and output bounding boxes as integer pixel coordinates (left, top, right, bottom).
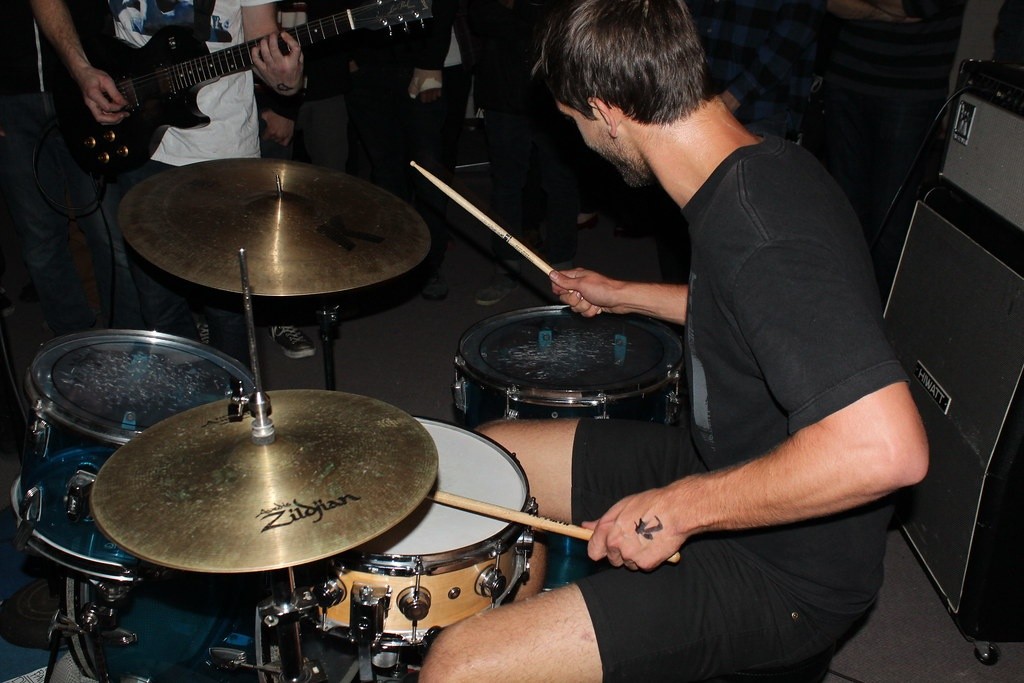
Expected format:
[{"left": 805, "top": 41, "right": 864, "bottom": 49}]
[{"left": 878, "top": 179, "right": 1024, "bottom": 645}]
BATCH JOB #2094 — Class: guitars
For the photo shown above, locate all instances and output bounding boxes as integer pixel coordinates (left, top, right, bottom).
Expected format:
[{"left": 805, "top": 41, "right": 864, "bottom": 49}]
[{"left": 54, "top": 0, "right": 435, "bottom": 183}]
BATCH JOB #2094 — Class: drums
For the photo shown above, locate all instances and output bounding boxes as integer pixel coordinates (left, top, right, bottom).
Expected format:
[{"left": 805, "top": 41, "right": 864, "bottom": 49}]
[
  {"left": 10, "top": 328, "right": 259, "bottom": 585},
  {"left": 453, "top": 304, "right": 687, "bottom": 455},
  {"left": 286, "top": 414, "right": 538, "bottom": 647}
]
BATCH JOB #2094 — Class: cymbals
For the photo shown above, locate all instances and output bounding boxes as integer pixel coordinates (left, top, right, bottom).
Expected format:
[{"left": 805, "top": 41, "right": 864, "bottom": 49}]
[
  {"left": 118, "top": 155, "right": 431, "bottom": 297},
  {"left": 91, "top": 388, "right": 440, "bottom": 573}
]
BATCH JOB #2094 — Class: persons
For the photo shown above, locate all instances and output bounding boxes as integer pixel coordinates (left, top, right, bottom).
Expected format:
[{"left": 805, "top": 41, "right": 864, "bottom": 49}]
[
  {"left": 1, "top": 0, "right": 1024, "bottom": 389},
  {"left": 416, "top": 0, "right": 930, "bottom": 683}
]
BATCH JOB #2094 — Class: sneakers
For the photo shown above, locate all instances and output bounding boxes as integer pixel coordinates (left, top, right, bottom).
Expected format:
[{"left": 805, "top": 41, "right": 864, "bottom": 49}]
[
  {"left": 421, "top": 271, "right": 447, "bottom": 301},
  {"left": 196, "top": 322, "right": 210, "bottom": 346},
  {"left": 475, "top": 273, "right": 519, "bottom": 305},
  {"left": 268, "top": 325, "right": 316, "bottom": 359}
]
